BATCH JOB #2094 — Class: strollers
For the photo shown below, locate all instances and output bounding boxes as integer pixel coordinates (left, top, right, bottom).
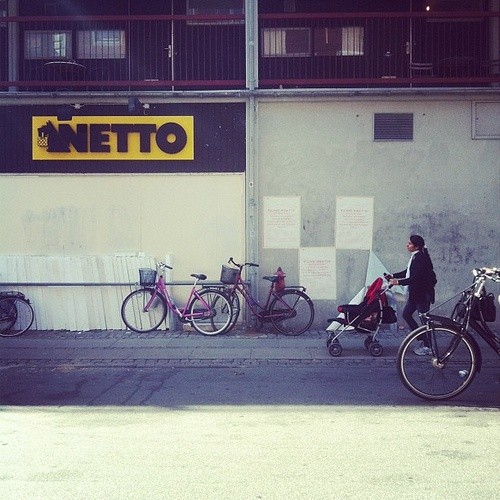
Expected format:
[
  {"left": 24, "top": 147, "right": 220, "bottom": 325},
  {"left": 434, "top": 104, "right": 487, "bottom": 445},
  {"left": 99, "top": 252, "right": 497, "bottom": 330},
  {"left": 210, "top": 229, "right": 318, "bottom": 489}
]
[{"left": 323, "top": 272, "right": 398, "bottom": 358}]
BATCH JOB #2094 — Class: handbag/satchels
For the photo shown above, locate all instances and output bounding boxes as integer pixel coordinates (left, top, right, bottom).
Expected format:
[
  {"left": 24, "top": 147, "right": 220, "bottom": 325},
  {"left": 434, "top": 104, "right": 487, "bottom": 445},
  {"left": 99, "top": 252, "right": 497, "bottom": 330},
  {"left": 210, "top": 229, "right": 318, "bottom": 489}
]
[
  {"left": 457, "top": 285, "right": 496, "bottom": 322},
  {"left": 382, "top": 306, "right": 398, "bottom": 324}
]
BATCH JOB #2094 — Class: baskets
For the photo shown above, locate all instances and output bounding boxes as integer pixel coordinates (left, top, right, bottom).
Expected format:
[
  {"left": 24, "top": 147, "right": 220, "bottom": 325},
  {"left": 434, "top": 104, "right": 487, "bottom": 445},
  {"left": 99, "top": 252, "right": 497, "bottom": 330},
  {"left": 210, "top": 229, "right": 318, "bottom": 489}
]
[
  {"left": 220, "top": 264, "right": 240, "bottom": 284},
  {"left": 139, "top": 267, "right": 157, "bottom": 285}
]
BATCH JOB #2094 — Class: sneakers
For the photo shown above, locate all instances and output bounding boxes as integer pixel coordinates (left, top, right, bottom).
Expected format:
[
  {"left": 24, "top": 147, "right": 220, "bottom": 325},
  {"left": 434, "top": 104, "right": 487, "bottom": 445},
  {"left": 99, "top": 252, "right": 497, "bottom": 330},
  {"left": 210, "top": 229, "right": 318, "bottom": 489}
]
[{"left": 412, "top": 340, "right": 436, "bottom": 355}]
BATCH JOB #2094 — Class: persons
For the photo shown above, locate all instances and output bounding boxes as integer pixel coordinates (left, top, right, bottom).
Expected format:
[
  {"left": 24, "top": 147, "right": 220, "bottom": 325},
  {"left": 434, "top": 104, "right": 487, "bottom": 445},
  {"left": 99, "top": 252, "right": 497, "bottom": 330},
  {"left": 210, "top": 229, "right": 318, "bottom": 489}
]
[{"left": 385, "top": 235, "right": 436, "bottom": 356}]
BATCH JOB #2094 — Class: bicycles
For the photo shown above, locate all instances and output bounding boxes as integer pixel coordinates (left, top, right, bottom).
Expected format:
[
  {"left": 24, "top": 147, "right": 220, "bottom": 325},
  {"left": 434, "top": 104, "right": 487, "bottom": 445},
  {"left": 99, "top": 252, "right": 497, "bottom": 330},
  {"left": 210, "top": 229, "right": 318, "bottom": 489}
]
[
  {"left": 0, "top": 290, "right": 34, "bottom": 337},
  {"left": 121, "top": 262, "right": 233, "bottom": 337},
  {"left": 395, "top": 268, "right": 500, "bottom": 401},
  {"left": 212, "top": 257, "right": 314, "bottom": 336}
]
